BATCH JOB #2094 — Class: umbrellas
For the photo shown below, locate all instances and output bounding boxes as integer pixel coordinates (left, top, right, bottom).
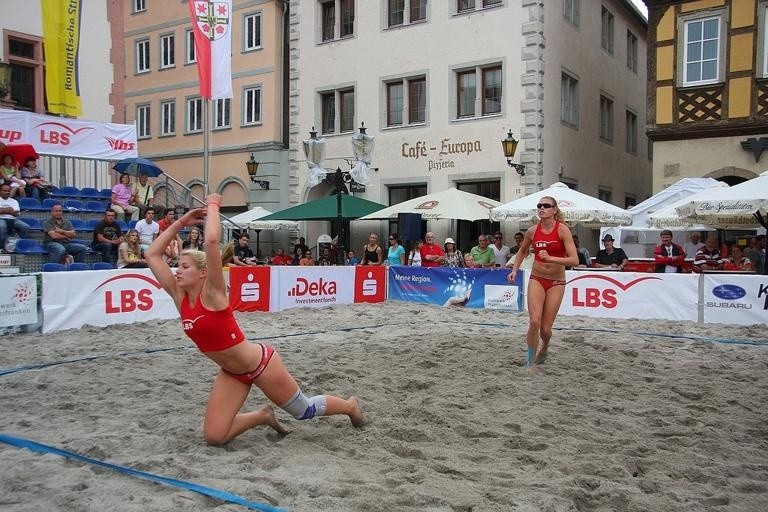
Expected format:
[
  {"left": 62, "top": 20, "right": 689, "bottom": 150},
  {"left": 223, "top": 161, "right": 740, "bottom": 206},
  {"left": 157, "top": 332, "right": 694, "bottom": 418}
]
[
  {"left": 219, "top": 207, "right": 299, "bottom": 258},
  {"left": 252, "top": 189, "right": 388, "bottom": 246},
  {"left": 0, "top": 142, "right": 40, "bottom": 171},
  {"left": 356, "top": 188, "right": 503, "bottom": 250},
  {"left": 490, "top": 182, "right": 634, "bottom": 231},
  {"left": 673, "top": 169, "right": 768, "bottom": 273},
  {"left": 645, "top": 182, "right": 731, "bottom": 253}
]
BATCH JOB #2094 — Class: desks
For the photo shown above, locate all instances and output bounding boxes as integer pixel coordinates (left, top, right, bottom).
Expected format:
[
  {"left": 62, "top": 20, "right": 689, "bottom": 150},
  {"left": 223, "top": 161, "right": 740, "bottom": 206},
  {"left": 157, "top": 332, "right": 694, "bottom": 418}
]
[{"left": 575, "top": 256, "right": 754, "bottom": 273}]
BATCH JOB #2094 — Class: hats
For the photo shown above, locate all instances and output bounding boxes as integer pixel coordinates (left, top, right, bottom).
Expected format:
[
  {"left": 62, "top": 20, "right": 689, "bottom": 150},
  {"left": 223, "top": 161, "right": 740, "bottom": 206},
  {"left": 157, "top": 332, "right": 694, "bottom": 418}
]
[
  {"left": 602, "top": 234, "right": 615, "bottom": 242},
  {"left": 443, "top": 237, "right": 455, "bottom": 246}
]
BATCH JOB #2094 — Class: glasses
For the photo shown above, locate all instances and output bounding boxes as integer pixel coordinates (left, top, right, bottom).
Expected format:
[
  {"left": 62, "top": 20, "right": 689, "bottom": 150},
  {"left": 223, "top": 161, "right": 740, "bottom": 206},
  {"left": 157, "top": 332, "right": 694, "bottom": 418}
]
[
  {"left": 537, "top": 203, "right": 554, "bottom": 209},
  {"left": 388, "top": 237, "right": 396, "bottom": 240}
]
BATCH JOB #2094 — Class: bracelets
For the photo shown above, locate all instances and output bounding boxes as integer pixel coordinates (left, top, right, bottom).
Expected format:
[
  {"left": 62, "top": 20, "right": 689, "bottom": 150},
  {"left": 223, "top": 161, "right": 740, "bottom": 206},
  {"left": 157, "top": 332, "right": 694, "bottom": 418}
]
[{"left": 206, "top": 201, "right": 220, "bottom": 207}]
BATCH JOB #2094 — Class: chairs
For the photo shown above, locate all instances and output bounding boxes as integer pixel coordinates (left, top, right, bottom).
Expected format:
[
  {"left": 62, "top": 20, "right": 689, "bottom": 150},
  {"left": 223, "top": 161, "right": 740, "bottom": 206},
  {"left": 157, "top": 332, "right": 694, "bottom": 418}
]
[{"left": 0, "top": 182, "right": 193, "bottom": 271}]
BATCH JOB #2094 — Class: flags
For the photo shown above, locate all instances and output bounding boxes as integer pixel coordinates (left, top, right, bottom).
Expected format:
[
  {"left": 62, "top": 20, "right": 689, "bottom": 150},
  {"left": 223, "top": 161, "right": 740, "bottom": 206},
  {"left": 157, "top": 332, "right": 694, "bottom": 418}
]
[{"left": 189, "top": 0, "right": 233, "bottom": 100}]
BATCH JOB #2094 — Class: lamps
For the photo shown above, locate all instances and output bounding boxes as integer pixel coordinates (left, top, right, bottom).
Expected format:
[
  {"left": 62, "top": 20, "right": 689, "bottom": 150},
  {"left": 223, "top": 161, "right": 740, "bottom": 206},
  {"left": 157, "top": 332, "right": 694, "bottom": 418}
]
[
  {"left": 499, "top": 127, "right": 528, "bottom": 175},
  {"left": 244, "top": 152, "right": 272, "bottom": 191}
]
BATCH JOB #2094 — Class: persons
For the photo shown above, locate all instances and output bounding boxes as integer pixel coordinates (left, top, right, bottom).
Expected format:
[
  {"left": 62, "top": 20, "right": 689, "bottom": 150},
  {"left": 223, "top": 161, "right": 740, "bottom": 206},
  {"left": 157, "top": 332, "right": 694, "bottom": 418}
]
[
  {"left": 1, "top": 154, "right": 201, "bottom": 272},
  {"left": 508, "top": 196, "right": 579, "bottom": 371},
  {"left": 653, "top": 230, "right": 766, "bottom": 273},
  {"left": 142, "top": 190, "right": 365, "bottom": 444},
  {"left": 567, "top": 235, "right": 628, "bottom": 271}
]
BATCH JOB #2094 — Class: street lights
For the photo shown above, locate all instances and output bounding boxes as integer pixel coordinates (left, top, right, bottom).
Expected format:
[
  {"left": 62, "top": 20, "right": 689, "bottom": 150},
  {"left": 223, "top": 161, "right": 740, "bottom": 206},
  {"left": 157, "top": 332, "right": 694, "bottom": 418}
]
[{"left": 304, "top": 124, "right": 373, "bottom": 262}]
